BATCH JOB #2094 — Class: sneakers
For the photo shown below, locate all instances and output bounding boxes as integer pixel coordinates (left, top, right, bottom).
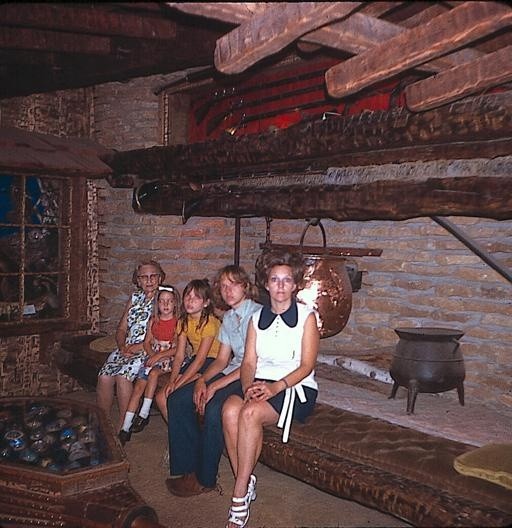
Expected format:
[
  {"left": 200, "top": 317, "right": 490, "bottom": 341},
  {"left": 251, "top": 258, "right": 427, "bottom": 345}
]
[
  {"left": 166, "top": 473, "right": 216, "bottom": 498},
  {"left": 118, "top": 429, "right": 132, "bottom": 446},
  {"left": 129, "top": 410, "right": 150, "bottom": 433}
]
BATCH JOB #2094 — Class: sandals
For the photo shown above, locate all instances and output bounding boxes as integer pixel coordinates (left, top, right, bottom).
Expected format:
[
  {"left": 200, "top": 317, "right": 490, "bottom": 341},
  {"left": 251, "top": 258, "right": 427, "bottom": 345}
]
[{"left": 225, "top": 474, "right": 256, "bottom": 527}]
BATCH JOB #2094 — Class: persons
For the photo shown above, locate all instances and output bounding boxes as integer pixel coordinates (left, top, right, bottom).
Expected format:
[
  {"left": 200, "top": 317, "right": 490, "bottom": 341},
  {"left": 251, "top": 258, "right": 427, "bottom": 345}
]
[
  {"left": 221, "top": 245, "right": 323, "bottom": 528},
  {"left": 114, "top": 285, "right": 181, "bottom": 447},
  {"left": 96, "top": 261, "right": 165, "bottom": 435},
  {"left": 166, "top": 264, "right": 266, "bottom": 498},
  {"left": 155, "top": 279, "right": 221, "bottom": 426}
]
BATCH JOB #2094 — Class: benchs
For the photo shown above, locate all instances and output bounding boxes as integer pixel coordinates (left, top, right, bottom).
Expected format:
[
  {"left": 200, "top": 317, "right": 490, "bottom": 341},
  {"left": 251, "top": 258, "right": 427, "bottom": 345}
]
[{"left": 61, "top": 335, "right": 512, "bottom": 528}]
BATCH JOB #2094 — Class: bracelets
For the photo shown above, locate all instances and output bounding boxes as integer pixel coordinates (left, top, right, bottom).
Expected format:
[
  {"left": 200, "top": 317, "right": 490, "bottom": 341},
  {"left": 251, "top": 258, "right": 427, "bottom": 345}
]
[{"left": 281, "top": 379, "right": 288, "bottom": 388}]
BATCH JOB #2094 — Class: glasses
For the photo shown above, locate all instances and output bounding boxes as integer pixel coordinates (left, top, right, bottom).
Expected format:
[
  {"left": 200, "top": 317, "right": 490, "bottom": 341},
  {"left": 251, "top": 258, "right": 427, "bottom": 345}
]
[{"left": 138, "top": 274, "right": 161, "bottom": 281}]
